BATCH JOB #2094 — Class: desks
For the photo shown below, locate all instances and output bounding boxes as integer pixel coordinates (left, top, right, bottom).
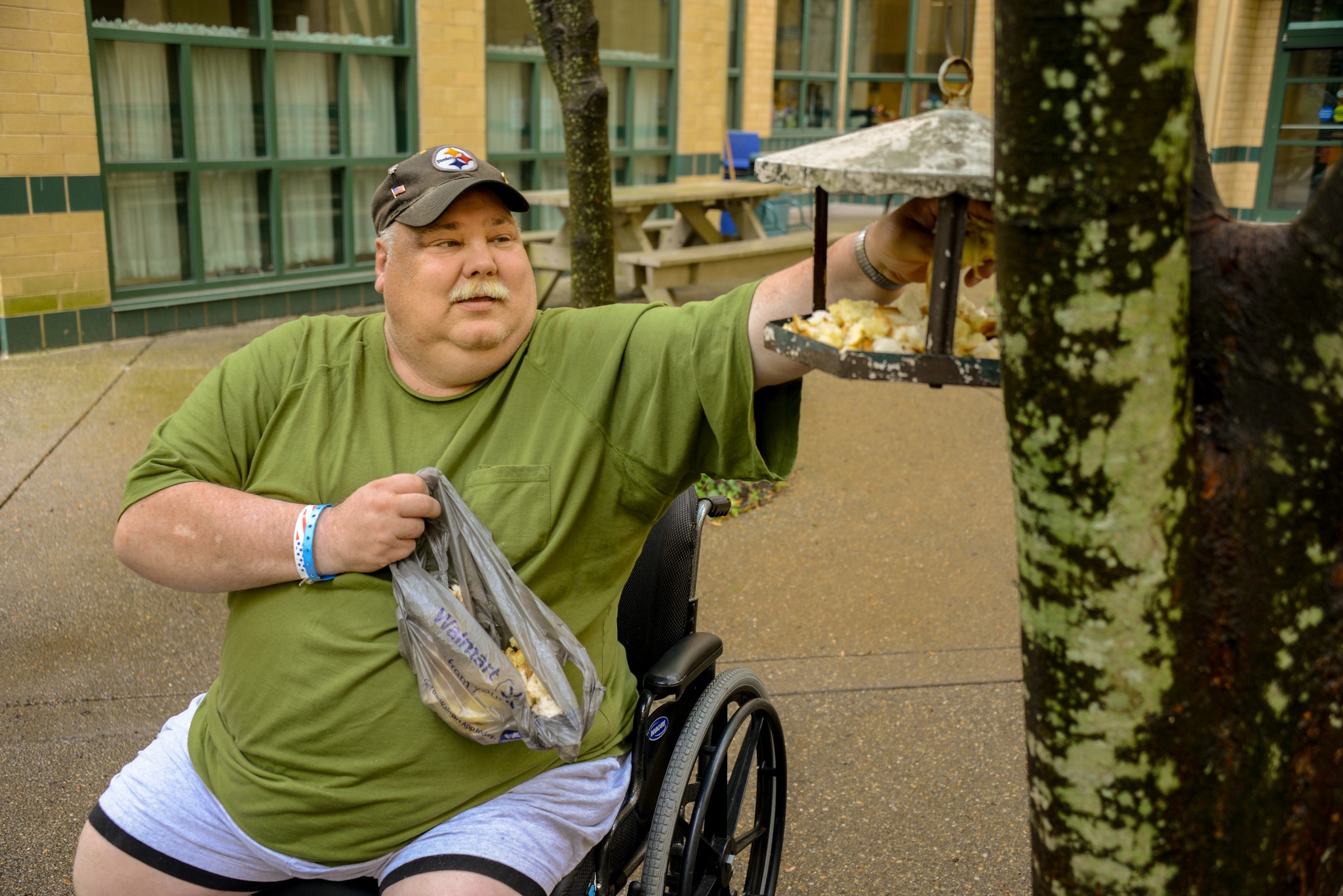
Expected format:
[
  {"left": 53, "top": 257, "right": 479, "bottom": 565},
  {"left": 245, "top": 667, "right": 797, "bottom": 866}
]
[{"left": 514, "top": 178, "right": 804, "bottom": 304}]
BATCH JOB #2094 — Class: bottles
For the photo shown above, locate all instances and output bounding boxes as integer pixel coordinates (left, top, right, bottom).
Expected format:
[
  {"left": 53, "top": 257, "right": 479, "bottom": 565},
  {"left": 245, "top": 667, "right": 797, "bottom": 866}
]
[{"left": 785, "top": 108, "right": 794, "bottom": 128}]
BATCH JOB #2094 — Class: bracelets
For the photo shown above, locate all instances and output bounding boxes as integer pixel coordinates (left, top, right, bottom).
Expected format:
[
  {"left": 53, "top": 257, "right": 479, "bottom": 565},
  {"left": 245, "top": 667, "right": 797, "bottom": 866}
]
[
  {"left": 854, "top": 221, "right": 904, "bottom": 291},
  {"left": 294, "top": 504, "right": 336, "bottom": 586}
]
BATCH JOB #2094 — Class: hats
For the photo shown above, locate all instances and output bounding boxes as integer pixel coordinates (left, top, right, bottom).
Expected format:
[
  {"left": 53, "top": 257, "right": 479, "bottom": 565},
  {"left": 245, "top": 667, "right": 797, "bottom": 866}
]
[{"left": 371, "top": 145, "right": 530, "bottom": 234}]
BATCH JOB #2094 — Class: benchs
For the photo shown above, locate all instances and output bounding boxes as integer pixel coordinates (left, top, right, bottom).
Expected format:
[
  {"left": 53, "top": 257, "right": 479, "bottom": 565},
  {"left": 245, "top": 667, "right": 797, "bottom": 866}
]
[
  {"left": 520, "top": 219, "right": 675, "bottom": 247},
  {"left": 617, "top": 231, "right": 846, "bottom": 286}
]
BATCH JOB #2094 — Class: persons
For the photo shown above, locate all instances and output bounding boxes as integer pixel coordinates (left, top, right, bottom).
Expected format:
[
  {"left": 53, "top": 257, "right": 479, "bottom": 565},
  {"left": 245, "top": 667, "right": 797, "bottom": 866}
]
[{"left": 71, "top": 144, "right": 995, "bottom": 896}]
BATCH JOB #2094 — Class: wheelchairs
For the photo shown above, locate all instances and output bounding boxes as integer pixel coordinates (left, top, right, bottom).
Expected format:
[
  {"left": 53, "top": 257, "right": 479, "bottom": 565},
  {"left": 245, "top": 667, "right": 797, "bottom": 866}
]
[{"left": 256, "top": 483, "right": 788, "bottom": 896}]
